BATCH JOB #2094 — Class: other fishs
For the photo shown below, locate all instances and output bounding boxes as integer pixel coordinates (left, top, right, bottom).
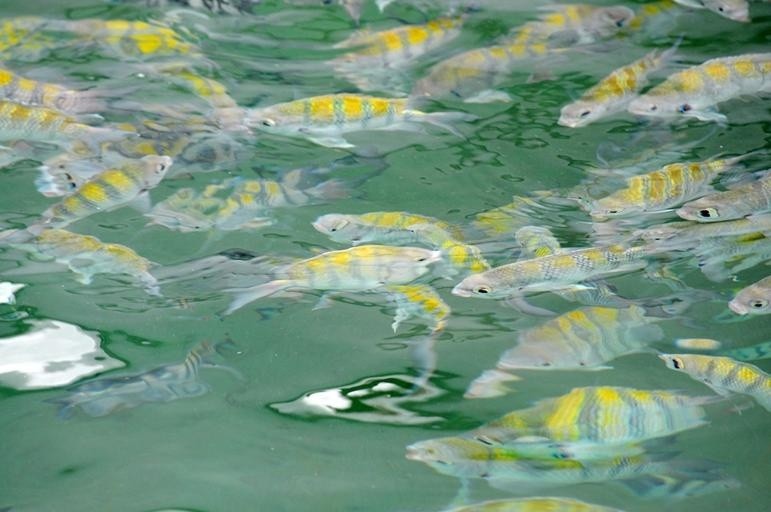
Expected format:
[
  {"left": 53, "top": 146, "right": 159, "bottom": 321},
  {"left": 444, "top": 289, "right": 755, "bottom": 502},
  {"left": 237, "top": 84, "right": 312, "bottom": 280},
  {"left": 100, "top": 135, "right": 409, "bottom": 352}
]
[{"left": 0, "top": 0, "right": 771, "bottom": 512}]
